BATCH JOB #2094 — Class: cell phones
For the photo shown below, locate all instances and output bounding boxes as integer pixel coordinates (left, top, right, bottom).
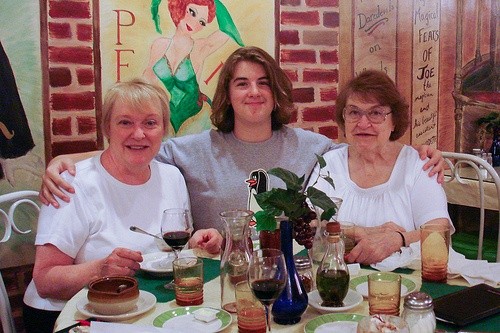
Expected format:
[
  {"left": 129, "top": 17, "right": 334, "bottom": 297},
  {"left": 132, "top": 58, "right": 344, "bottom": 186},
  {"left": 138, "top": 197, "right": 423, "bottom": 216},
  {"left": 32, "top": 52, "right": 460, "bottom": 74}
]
[{"left": 432, "top": 282, "right": 500, "bottom": 327}]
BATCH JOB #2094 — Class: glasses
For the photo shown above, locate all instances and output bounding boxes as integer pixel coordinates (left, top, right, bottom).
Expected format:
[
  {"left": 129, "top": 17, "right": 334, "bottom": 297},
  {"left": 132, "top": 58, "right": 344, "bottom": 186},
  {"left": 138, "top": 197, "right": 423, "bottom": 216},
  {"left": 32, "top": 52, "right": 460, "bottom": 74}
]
[{"left": 343, "top": 105, "right": 393, "bottom": 124}]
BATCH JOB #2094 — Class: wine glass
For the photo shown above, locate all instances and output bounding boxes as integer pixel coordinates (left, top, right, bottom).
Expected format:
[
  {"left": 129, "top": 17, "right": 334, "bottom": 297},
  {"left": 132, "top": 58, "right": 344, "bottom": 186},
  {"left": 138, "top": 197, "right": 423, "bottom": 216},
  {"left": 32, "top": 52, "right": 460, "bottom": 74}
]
[
  {"left": 155, "top": 233, "right": 175, "bottom": 268},
  {"left": 160, "top": 207, "right": 191, "bottom": 258},
  {"left": 246, "top": 248, "right": 288, "bottom": 333}
]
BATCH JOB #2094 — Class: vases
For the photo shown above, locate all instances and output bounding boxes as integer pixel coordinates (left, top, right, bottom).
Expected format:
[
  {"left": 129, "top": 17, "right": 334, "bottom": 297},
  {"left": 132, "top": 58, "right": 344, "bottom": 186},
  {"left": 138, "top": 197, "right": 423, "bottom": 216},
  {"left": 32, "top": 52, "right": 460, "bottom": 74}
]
[{"left": 271, "top": 221, "right": 308, "bottom": 326}]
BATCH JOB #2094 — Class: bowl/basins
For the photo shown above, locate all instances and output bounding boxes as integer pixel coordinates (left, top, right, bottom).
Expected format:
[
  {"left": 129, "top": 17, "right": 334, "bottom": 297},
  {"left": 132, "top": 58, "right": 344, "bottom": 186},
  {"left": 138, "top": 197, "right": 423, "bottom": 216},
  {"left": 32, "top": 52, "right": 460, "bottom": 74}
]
[{"left": 87, "top": 274, "right": 140, "bottom": 316}]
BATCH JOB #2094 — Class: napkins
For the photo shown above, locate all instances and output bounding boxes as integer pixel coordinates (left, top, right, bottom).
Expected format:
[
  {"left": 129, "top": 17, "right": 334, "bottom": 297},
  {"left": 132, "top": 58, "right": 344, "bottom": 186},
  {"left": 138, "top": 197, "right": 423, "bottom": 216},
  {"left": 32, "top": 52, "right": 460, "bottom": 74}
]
[{"left": 371, "top": 240, "right": 500, "bottom": 286}]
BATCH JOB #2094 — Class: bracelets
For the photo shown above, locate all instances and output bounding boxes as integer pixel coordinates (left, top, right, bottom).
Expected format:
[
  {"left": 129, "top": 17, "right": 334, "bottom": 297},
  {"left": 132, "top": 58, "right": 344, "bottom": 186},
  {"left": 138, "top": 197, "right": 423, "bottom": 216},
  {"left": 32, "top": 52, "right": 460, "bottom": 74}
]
[{"left": 396, "top": 231, "right": 406, "bottom": 247}]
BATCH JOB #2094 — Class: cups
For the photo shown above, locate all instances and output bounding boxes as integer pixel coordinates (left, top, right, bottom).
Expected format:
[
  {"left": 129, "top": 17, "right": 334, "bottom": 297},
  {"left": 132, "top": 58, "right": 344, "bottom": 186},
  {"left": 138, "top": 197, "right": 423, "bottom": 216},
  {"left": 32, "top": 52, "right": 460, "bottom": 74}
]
[
  {"left": 367, "top": 272, "right": 401, "bottom": 316},
  {"left": 420, "top": 223, "right": 451, "bottom": 283},
  {"left": 172, "top": 257, "right": 204, "bottom": 307},
  {"left": 339, "top": 220, "right": 356, "bottom": 265},
  {"left": 234, "top": 280, "right": 267, "bottom": 333},
  {"left": 357, "top": 314, "right": 411, "bottom": 333}
]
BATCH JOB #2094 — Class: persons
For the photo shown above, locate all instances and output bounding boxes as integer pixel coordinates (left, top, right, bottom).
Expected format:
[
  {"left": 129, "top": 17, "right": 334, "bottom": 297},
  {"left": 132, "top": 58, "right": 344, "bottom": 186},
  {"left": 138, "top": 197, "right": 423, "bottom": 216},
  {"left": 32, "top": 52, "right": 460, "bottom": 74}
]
[
  {"left": 38, "top": 46, "right": 446, "bottom": 238},
  {"left": 301, "top": 70, "right": 455, "bottom": 264},
  {"left": 22, "top": 79, "right": 223, "bottom": 333}
]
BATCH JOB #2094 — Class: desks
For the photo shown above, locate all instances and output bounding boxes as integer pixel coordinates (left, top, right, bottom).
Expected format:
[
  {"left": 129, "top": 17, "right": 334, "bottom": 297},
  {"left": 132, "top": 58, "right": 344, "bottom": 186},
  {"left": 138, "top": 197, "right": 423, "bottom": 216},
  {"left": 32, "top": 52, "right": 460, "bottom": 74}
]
[
  {"left": 53, "top": 240, "right": 500, "bottom": 333},
  {"left": 440, "top": 168, "right": 500, "bottom": 211}
]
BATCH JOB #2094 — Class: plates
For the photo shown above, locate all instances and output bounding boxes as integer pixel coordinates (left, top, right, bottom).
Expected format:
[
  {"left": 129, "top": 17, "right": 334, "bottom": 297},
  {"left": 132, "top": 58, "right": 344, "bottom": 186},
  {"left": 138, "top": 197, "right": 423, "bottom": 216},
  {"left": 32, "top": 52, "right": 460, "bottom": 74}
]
[
  {"left": 303, "top": 313, "right": 367, "bottom": 333},
  {"left": 307, "top": 288, "right": 363, "bottom": 314},
  {"left": 76, "top": 290, "right": 157, "bottom": 322},
  {"left": 152, "top": 306, "right": 234, "bottom": 333},
  {"left": 138, "top": 251, "right": 198, "bottom": 273},
  {"left": 349, "top": 272, "right": 417, "bottom": 298}
]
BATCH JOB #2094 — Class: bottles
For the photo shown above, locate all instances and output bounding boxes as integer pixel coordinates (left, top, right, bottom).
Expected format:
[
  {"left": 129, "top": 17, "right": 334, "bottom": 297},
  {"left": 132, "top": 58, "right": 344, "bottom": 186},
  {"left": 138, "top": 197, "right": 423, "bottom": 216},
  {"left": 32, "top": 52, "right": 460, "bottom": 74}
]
[
  {"left": 219, "top": 226, "right": 254, "bottom": 284},
  {"left": 295, "top": 255, "right": 313, "bottom": 293},
  {"left": 401, "top": 292, "right": 436, "bottom": 333},
  {"left": 218, "top": 207, "right": 254, "bottom": 314},
  {"left": 472, "top": 148, "right": 492, "bottom": 169},
  {"left": 271, "top": 220, "right": 308, "bottom": 325},
  {"left": 316, "top": 223, "right": 350, "bottom": 307}
]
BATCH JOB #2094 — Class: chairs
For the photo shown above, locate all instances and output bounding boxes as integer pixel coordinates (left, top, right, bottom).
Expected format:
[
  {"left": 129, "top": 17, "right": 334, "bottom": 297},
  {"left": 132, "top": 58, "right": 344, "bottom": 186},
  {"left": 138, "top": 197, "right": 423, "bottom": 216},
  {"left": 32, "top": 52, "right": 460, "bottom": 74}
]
[
  {"left": 0, "top": 190, "right": 43, "bottom": 333},
  {"left": 439, "top": 152, "right": 500, "bottom": 262}
]
